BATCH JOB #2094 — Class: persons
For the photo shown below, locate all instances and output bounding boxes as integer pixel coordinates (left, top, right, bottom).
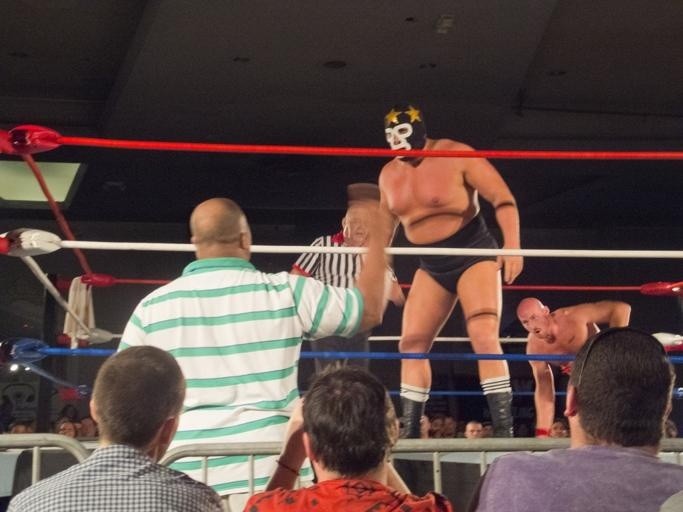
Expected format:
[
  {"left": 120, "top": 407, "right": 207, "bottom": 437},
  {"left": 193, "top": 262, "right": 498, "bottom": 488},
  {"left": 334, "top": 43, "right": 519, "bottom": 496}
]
[
  {"left": 516, "top": 298, "right": 631, "bottom": 438},
  {"left": 116, "top": 185, "right": 384, "bottom": 510},
  {"left": 243, "top": 368, "right": 452, "bottom": 512},
  {"left": 552, "top": 419, "right": 569, "bottom": 436},
  {"left": 0, "top": 405, "right": 97, "bottom": 448},
  {"left": 377, "top": 95, "right": 523, "bottom": 440},
  {"left": 419, "top": 413, "right": 494, "bottom": 438},
  {"left": 4, "top": 346, "right": 222, "bottom": 512},
  {"left": 290, "top": 204, "right": 407, "bottom": 376},
  {"left": 467, "top": 327, "right": 683, "bottom": 512}
]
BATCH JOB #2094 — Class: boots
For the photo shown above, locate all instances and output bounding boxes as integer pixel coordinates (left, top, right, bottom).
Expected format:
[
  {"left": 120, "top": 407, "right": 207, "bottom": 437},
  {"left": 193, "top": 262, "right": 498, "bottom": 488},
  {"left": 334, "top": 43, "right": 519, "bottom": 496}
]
[
  {"left": 486, "top": 392, "right": 515, "bottom": 438},
  {"left": 397, "top": 395, "right": 425, "bottom": 438}
]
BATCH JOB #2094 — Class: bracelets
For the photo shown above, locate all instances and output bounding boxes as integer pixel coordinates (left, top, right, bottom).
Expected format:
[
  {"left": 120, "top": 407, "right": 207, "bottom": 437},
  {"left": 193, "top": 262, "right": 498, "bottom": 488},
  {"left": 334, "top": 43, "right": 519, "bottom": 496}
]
[
  {"left": 276, "top": 461, "right": 302, "bottom": 477},
  {"left": 533, "top": 429, "right": 552, "bottom": 438}
]
